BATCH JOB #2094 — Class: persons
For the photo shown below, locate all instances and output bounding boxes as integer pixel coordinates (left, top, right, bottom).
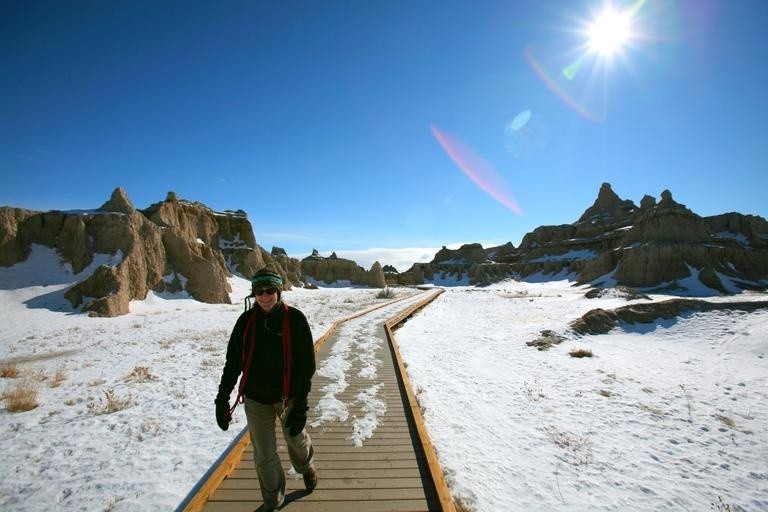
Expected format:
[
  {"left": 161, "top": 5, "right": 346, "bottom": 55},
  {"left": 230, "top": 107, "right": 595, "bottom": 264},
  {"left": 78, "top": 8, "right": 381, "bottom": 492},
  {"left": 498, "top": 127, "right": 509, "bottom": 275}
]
[{"left": 214, "top": 262, "right": 317, "bottom": 512}]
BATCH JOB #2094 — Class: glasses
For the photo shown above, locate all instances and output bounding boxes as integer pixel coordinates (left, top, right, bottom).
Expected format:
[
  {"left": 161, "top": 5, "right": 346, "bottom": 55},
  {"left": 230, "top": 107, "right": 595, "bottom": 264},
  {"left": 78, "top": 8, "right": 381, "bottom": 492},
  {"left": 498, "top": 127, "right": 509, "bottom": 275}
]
[{"left": 254, "top": 288, "right": 276, "bottom": 295}]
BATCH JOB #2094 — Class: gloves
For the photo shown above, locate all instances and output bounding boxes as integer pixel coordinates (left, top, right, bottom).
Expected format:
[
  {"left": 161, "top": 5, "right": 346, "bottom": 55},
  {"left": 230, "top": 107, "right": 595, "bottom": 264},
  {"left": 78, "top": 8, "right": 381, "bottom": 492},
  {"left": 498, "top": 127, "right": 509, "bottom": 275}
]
[
  {"left": 215, "top": 400, "right": 230, "bottom": 430},
  {"left": 303, "top": 464, "right": 317, "bottom": 491},
  {"left": 286, "top": 400, "right": 307, "bottom": 436}
]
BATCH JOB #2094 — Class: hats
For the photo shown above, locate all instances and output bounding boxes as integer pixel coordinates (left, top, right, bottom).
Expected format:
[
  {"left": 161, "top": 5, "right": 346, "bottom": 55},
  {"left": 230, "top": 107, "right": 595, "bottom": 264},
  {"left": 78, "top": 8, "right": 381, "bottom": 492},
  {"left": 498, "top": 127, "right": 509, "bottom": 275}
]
[{"left": 248, "top": 268, "right": 282, "bottom": 300}]
